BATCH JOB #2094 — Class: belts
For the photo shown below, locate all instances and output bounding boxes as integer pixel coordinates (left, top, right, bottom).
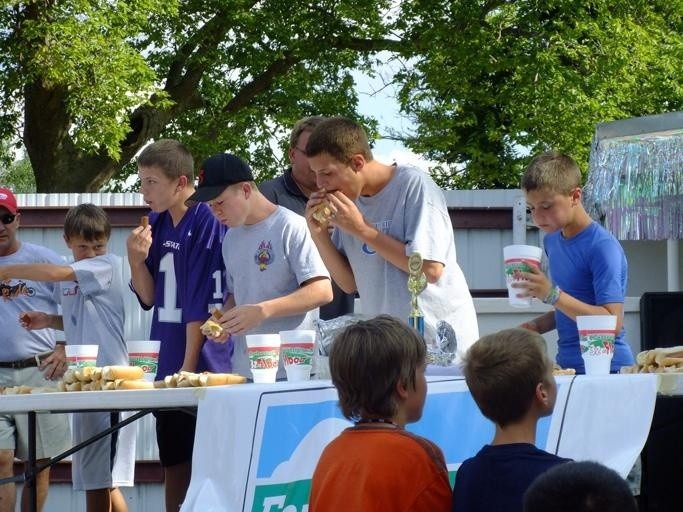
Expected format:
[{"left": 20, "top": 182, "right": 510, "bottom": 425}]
[{"left": 0, "top": 351, "right": 55, "bottom": 370}]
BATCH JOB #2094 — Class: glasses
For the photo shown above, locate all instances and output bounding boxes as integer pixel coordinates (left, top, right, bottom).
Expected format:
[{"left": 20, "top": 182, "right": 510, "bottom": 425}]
[
  {"left": 295, "top": 146, "right": 307, "bottom": 155},
  {"left": 1, "top": 215, "right": 16, "bottom": 227}
]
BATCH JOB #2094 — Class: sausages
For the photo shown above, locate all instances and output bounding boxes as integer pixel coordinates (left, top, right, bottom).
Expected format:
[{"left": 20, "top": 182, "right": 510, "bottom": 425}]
[
  {"left": 211, "top": 308, "right": 225, "bottom": 325},
  {"left": 140, "top": 215, "right": 149, "bottom": 228}
]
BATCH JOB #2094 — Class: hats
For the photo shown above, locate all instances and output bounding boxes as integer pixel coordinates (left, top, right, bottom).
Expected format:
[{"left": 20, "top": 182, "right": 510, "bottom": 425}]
[
  {"left": 0, "top": 186, "right": 17, "bottom": 216},
  {"left": 185, "top": 154, "right": 254, "bottom": 209}
]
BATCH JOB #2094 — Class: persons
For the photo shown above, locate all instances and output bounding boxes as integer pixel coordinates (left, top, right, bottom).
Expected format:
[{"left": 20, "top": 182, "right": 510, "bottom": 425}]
[
  {"left": 510, "top": 151, "right": 643, "bottom": 512},
  {"left": 124, "top": 140, "right": 236, "bottom": 511},
  {"left": 262, "top": 117, "right": 358, "bottom": 318},
  {"left": 456, "top": 328, "right": 575, "bottom": 512},
  {"left": 304, "top": 120, "right": 479, "bottom": 379},
  {"left": 184, "top": 153, "right": 332, "bottom": 390},
  {"left": 0, "top": 188, "right": 68, "bottom": 512},
  {"left": 2, "top": 203, "right": 139, "bottom": 512},
  {"left": 522, "top": 460, "right": 639, "bottom": 512},
  {"left": 308, "top": 317, "right": 454, "bottom": 512}
]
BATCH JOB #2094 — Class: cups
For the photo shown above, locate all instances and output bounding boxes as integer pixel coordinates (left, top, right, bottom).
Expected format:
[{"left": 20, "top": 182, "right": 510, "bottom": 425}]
[
  {"left": 64, "top": 343, "right": 100, "bottom": 389},
  {"left": 576, "top": 315, "right": 617, "bottom": 376},
  {"left": 503, "top": 244, "right": 542, "bottom": 309},
  {"left": 124, "top": 340, "right": 161, "bottom": 389},
  {"left": 279, "top": 329, "right": 317, "bottom": 387},
  {"left": 244, "top": 333, "right": 282, "bottom": 386}
]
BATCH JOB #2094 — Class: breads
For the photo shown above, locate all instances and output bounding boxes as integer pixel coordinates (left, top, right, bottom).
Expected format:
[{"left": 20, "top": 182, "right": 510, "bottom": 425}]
[
  {"left": 620, "top": 353, "right": 662, "bottom": 373},
  {"left": 199, "top": 374, "right": 245, "bottom": 386},
  {"left": 165, "top": 371, "right": 210, "bottom": 388},
  {"left": 312, "top": 193, "right": 334, "bottom": 228},
  {"left": 56, "top": 366, "right": 101, "bottom": 391},
  {"left": 656, "top": 346, "right": 682, "bottom": 366},
  {"left": 201, "top": 321, "right": 221, "bottom": 337},
  {"left": 114, "top": 381, "right": 153, "bottom": 390},
  {"left": 2, "top": 385, "right": 57, "bottom": 395},
  {"left": 100, "top": 365, "right": 142, "bottom": 381}
]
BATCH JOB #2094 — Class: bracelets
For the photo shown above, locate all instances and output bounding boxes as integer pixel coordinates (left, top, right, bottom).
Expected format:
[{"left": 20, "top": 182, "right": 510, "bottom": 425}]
[{"left": 542, "top": 283, "right": 561, "bottom": 304}]
